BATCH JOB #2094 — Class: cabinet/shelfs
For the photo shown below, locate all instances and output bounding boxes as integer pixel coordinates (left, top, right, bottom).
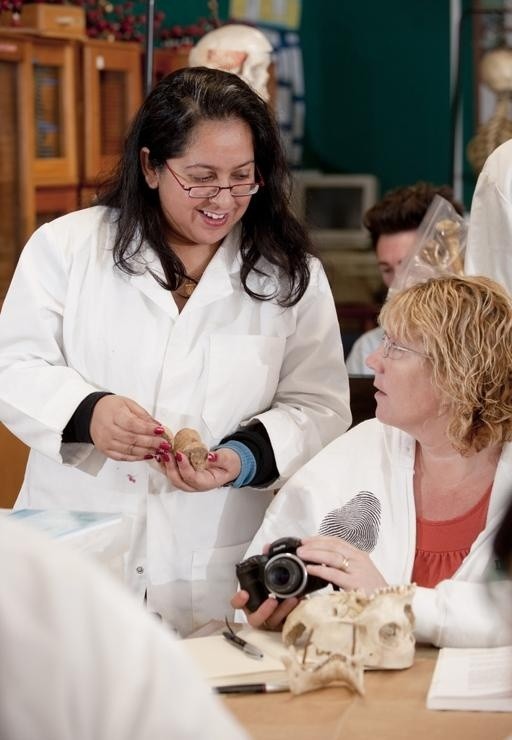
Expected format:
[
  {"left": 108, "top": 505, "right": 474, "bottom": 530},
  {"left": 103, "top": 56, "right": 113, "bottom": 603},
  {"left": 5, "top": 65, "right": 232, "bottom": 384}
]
[{"left": 1, "top": 26, "right": 144, "bottom": 289}]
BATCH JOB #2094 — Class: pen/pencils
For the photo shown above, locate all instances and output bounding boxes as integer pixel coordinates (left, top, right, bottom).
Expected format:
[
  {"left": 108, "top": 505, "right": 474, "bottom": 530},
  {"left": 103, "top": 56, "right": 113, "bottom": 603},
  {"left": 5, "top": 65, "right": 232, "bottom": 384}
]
[{"left": 222, "top": 631, "right": 263, "bottom": 658}]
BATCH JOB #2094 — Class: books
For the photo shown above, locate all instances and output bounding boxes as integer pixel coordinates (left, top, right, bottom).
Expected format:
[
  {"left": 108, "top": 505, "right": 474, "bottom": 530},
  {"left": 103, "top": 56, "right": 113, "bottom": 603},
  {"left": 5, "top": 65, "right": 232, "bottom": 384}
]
[
  {"left": 175, "top": 630, "right": 307, "bottom": 686},
  {"left": 422, "top": 648, "right": 511, "bottom": 714}
]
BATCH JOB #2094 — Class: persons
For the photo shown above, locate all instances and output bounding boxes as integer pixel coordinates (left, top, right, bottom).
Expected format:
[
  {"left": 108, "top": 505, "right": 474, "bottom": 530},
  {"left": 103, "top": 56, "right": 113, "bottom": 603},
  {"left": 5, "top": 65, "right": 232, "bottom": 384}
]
[
  {"left": 342, "top": 181, "right": 468, "bottom": 381},
  {"left": 229, "top": 279, "right": 512, "bottom": 649},
  {"left": 1, "top": 64, "right": 353, "bottom": 638},
  {"left": 0, "top": 508, "right": 239, "bottom": 738}
]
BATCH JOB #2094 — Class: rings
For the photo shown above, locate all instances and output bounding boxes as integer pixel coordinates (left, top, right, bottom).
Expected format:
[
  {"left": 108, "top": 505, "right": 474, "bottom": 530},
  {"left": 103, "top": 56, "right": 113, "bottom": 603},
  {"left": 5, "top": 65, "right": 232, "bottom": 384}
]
[{"left": 341, "top": 557, "right": 350, "bottom": 569}]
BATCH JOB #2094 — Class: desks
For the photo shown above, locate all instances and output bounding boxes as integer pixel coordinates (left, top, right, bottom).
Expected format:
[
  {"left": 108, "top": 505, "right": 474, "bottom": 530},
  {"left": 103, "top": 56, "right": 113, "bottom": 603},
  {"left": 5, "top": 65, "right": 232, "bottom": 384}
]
[{"left": 179, "top": 627, "right": 511, "bottom": 740}]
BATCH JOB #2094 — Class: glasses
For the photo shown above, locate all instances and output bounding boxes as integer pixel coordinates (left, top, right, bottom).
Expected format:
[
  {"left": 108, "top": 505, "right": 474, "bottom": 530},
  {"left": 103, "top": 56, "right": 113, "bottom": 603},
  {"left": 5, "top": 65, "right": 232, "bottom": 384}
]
[
  {"left": 162, "top": 157, "right": 266, "bottom": 199},
  {"left": 374, "top": 331, "right": 434, "bottom": 362}
]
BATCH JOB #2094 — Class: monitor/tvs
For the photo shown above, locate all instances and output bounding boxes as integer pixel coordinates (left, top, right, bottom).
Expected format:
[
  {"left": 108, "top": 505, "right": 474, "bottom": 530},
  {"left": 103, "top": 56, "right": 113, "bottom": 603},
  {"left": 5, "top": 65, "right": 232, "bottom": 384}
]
[{"left": 294, "top": 174, "right": 378, "bottom": 251}]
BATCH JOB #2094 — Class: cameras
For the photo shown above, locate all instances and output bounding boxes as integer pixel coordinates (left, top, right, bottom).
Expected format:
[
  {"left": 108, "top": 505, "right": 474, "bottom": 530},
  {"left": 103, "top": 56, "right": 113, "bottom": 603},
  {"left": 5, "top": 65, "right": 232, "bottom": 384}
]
[{"left": 236, "top": 537, "right": 332, "bottom": 614}]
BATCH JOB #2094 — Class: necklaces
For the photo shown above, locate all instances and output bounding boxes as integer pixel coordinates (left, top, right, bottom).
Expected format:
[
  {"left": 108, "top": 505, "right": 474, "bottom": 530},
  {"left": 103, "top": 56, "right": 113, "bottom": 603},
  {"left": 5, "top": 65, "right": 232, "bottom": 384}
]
[{"left": 173, "top": 274, "right": 203, "bottom": 299}]
